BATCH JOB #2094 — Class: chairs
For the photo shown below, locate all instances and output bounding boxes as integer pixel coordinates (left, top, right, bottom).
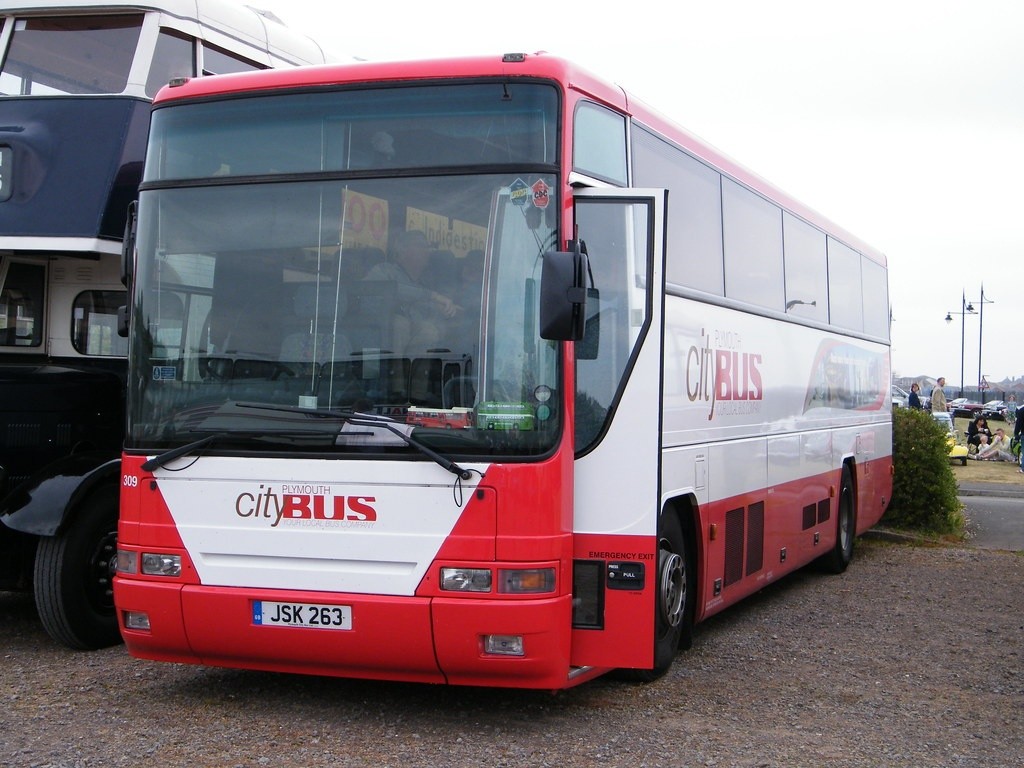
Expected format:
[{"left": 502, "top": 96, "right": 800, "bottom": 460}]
[
  {"left": 964, "top": 431, "right": 980, "bottom": 454},
  {"left": 271, "top": 282, "right": 355, "bottom": 405}
]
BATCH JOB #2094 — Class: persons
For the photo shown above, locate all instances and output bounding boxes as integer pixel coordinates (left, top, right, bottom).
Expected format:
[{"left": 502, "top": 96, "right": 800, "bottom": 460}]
[
  {"left": 1014, "top": 405, "right": 1024, "bottom": 474},
  {"left": 367, "top": 229, "right": 466, "bottom": 398},
  {"left": 968, "top": 429, "right": 1014, "bottom": 461},
  {"left": 968, "top": 416, "right": 992, "bottom": 454},
  {"left": 931, "top": 377, "right": 950, "bottom": 412},
  {"left": 909, "top": 383, "right": 922, "bottom": 408},
  {"left": 978, "top": 436, "right": 989, "bottom": 453}
]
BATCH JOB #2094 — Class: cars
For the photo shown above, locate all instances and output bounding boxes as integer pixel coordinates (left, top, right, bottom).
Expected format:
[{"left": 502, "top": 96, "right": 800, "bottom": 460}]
[
  {"left": 892, "top": 385, "right": 926, "bottom": 408},
  {"left": 946, "top": 398, "right": 1005, "bottom": 419}
]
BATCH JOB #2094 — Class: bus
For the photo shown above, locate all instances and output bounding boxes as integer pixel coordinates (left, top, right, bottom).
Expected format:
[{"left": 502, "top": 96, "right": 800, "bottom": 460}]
[{"left": 1, "top": 0, "right": 896, "bottom": 691}]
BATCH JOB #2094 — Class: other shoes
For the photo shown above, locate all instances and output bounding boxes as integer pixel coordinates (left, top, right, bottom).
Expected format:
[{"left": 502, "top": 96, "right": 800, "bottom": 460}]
[
  {"left": 976, "top": 454, "right": 981, "bottom": 460},
  {"left": 968, "top": 454, "right": 975, "bottom": 459}
]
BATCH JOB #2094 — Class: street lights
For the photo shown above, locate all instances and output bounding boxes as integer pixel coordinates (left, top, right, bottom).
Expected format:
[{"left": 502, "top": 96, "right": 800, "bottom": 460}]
[
  {"left": 967, "top": 282, "right": 995, "bottom": 391},
  {"left": 945, "top": 287, "right": 978, "bottom": 398}
]
